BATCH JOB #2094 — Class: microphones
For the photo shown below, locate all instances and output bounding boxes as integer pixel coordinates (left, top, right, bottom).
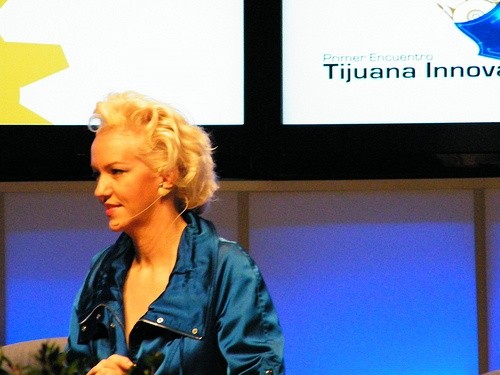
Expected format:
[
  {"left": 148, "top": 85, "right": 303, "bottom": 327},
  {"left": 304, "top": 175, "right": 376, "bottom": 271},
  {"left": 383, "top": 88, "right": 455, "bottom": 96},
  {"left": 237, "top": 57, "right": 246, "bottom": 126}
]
[{"left": 112, "top": 187, "right": 175, "bottom": 226}]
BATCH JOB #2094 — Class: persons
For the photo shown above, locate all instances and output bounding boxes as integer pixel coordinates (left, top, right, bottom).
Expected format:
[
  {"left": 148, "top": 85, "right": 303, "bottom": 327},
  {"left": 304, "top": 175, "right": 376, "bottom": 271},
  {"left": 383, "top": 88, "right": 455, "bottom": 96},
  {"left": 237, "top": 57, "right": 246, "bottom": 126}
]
[{"left": 63, "top": 91, "right": 286, "bottom": 375}]
